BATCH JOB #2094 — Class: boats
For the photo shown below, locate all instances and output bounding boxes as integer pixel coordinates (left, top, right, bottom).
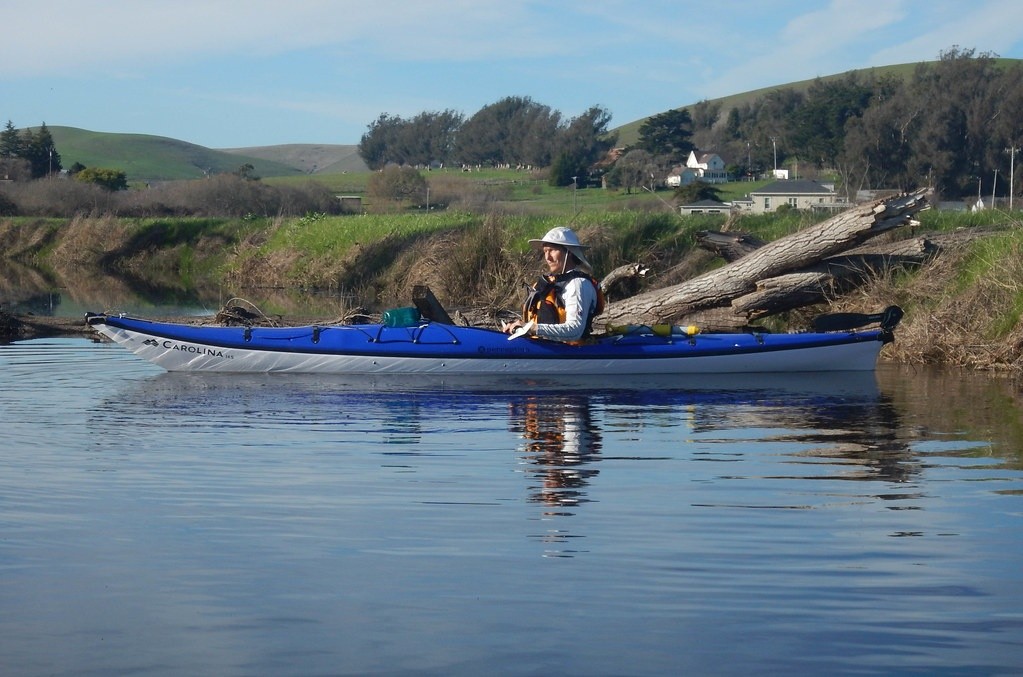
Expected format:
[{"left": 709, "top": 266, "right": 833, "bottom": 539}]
[{"left": 86, "top": 305, "right": 903, "bottom": 375}]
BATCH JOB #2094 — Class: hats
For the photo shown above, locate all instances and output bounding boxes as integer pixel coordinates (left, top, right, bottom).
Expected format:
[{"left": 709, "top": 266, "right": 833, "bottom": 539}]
[{"left": 527, "top": 227, "right": 592, "bottom": 274}]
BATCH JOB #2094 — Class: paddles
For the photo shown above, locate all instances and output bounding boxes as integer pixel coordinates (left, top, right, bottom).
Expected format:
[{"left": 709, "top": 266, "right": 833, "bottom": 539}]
[{"left": 501, "top": 318, "right": 534, "bottom": 341}]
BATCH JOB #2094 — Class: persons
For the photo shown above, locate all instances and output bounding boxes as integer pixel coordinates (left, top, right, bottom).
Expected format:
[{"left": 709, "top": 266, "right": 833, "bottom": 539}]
[{"left": 503, "top": 227, "right": 597, "bottom": 346}]
